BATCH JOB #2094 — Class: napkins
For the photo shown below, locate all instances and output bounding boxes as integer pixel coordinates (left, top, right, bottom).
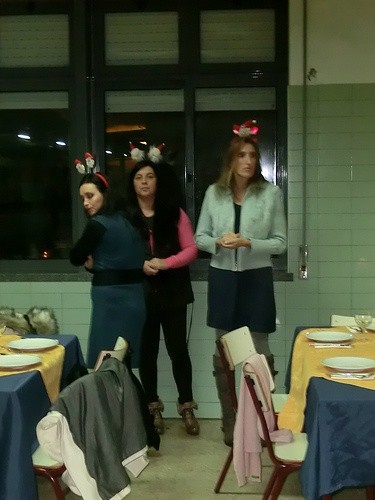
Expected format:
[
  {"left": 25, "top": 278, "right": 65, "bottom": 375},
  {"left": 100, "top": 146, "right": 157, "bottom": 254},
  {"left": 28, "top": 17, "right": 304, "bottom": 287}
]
[
  {"left": 314, "top": 342, "right": 351, "bottom": 349},
  {"left": 345, "top": 326, "right": 368, "bottom": 334},
  {"left": 330, "top": 371, "right": 375, "bottom": 381}
]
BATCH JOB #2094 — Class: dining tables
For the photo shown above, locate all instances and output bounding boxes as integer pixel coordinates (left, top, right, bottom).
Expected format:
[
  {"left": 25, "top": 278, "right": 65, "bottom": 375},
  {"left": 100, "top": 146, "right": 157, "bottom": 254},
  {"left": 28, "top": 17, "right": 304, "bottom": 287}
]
[{"left": 275, "top": 325, "right": 375, "bottom": 500}]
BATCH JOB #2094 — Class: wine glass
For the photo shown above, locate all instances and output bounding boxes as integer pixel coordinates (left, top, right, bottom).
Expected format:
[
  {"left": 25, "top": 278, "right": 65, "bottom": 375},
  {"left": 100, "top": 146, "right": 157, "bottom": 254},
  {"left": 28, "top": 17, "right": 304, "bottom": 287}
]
[{"left": 355, "top": 312, "right": 372, "bottom": 342}]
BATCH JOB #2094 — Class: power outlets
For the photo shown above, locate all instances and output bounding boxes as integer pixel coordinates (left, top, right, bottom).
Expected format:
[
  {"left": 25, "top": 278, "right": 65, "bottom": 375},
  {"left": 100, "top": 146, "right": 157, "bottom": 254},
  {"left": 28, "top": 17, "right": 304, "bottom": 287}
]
[{"left": 298, "top": 265, "right": 308, "bottom": 279}]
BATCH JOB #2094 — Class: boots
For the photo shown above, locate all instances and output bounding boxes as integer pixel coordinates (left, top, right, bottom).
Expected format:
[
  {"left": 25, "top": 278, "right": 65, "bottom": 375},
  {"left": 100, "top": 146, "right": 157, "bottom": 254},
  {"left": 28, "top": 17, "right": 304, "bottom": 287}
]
[
  {"left": 212, "top": 354, "right": 238, "bottom": 447},
  {"left": 176, "top": 396, "right": 201, "bottom": 435},
  {"left": 261, "top": 354, "right": 279, "bottom": 446},
  {"left": 149, "top": 399, "right": 165, "bottom": 435}
]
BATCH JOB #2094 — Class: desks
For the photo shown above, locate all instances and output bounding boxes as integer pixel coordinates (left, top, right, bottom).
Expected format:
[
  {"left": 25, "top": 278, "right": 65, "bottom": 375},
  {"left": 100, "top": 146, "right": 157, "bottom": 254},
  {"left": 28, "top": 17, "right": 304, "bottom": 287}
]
[{"left": 0, "top": 334, "right": 89, "bottom": 500}]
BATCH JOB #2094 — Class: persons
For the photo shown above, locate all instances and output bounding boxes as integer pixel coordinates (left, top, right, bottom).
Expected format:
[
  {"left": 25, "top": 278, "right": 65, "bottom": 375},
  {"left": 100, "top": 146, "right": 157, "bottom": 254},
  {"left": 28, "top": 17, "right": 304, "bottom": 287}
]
[
  {"left": 69, "top": 171, "right": 162, "bottom": 451},
  {"left": 193, "top": 134, "right": 288, "bottom": 447},
  {"left": 115, "top": 159, "right": 200, "bottom": 436}
]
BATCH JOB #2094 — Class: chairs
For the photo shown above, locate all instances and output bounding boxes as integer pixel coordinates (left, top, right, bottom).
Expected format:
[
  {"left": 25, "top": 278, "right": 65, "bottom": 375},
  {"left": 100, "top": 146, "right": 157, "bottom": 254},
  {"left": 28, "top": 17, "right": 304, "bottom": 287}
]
[
  {"left": 214, "top": 327, "right": 289, "bottom": 495},
  {"left": 232, "top": 354, "right": 309, "bottom": 500},
  {"left": 330, "top": 314, "right": 375, "bottom": 327},
  {"left": 29, "top": 336, "right": 150, "bottom": 500}
]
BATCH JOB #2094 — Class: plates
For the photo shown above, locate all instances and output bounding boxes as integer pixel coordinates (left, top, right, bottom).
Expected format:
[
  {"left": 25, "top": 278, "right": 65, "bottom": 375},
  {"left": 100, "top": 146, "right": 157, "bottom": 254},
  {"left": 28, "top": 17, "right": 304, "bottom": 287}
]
[
  {"left": 366, "top": 326, "right": 375, "bottom": 331},
  {"left": 7, "top": 338, "right": 59, "bottom": 352},
  {"left": 320, "top": 357, "right": 375, "bottom": 372},
  {"left": 305, "top": 331, "right": 354, "bottom": 344},
  {"left": 0, "top": 354, "right": 41, "bottom": 369}
]
